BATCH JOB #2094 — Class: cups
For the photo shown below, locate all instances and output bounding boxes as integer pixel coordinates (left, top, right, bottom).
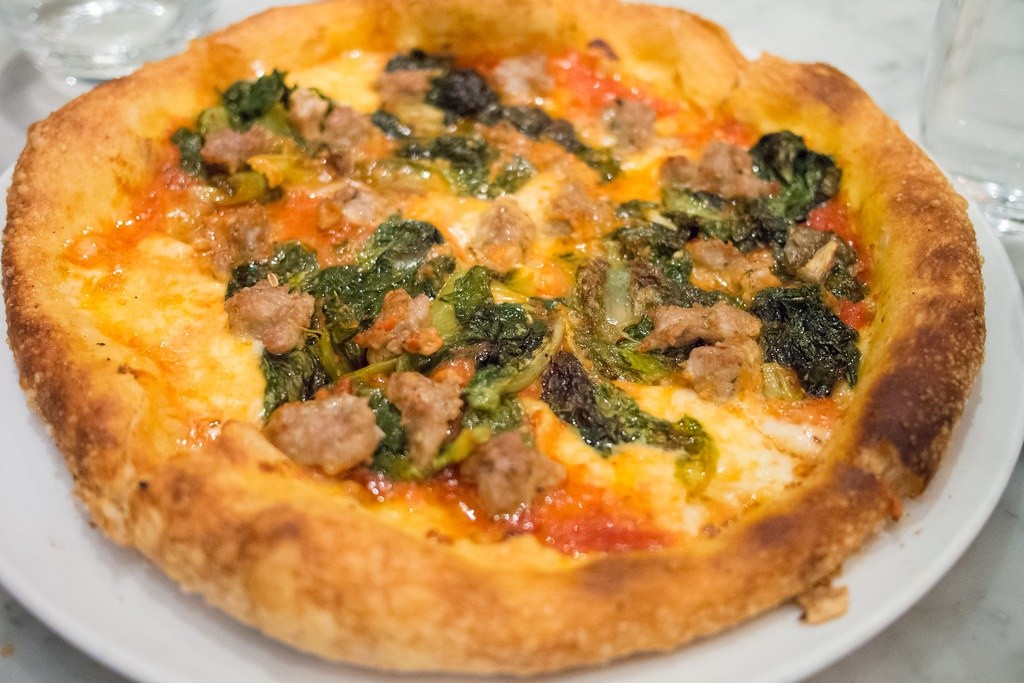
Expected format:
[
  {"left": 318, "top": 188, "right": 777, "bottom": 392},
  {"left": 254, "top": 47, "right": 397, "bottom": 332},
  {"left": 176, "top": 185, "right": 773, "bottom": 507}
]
[
  {"left": 919, "top": 0, "right": 1024, "bottom": 234},
  {"left": 0, "top": 0, "right": 211, "bottom": 81}
]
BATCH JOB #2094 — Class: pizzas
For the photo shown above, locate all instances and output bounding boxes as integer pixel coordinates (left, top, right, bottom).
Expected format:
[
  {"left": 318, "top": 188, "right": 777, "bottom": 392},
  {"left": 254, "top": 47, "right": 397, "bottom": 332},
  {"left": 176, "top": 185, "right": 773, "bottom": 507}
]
[{"left": 0, "top": 0, "right": 987, "bottom": 672}]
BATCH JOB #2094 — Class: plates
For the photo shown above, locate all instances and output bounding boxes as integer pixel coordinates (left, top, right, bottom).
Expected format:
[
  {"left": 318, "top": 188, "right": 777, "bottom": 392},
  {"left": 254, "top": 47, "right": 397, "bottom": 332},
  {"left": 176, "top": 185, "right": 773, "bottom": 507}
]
[{"left": 0, "top": 155, "right": 1024, "bottom": 683}]
